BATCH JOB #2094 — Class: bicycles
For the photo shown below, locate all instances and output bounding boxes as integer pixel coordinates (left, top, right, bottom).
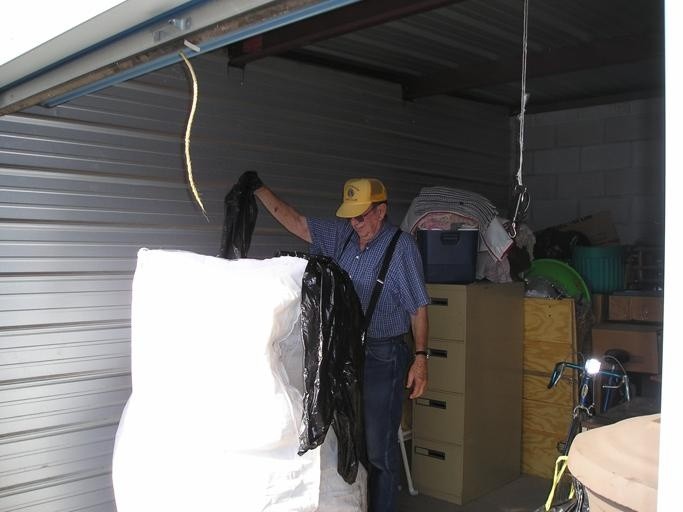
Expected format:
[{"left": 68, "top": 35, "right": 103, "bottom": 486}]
[{"left": 534, "top": 349, "right": 631, "bottom": 512}]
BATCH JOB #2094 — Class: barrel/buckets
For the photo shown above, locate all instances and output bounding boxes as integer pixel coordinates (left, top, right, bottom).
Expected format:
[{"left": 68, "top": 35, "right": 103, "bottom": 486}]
[{"left": 573, "top": 241, "right": 626, "bottom": 293}]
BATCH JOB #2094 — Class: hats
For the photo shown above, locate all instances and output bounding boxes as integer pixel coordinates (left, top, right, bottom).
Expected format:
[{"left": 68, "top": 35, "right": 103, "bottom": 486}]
[{"left": 335, "top": 178, "right": 387, "bottom": 218}]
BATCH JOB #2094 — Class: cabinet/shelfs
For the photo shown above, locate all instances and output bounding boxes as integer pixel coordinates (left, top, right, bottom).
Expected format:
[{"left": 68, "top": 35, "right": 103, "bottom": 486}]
[{"left": 406, "top": 283, "right": 526, "bottom": 506}]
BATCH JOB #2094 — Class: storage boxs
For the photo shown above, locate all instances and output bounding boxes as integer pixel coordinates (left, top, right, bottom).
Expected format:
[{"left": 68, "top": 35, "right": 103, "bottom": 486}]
[{"left": 562, "top": 210, "right": 663, "bottom": 380}]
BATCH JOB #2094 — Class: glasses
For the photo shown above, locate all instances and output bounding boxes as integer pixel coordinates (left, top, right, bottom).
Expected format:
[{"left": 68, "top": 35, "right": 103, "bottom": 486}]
[{"left": 348, "top": 202, "right": 384, "bottom": 222}]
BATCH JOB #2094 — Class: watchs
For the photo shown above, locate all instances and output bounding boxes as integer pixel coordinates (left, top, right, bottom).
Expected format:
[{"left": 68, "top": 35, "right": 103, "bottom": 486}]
[{"left": 416, "top": 350, "right": 431, "bottom": 360}]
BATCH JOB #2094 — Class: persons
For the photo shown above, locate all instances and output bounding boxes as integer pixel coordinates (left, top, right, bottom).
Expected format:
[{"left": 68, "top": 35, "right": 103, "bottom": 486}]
[{"left": 242, "top": 171, "right": 434, "bottom": 512}]
